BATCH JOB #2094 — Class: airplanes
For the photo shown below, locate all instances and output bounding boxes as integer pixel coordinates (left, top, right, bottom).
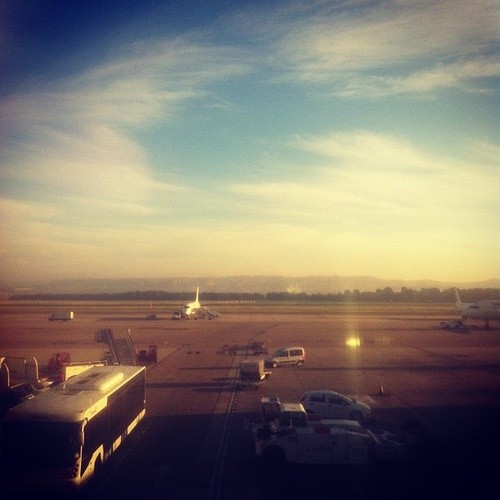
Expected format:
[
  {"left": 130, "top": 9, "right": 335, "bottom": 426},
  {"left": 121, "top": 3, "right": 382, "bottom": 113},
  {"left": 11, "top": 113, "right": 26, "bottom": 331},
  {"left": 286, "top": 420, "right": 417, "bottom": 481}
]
[
  {"left": 454, "top": 289, "right": 500, "bottom": 329},
  {"left": 181, "top": 286, "right": 202, "bottom": 320}
]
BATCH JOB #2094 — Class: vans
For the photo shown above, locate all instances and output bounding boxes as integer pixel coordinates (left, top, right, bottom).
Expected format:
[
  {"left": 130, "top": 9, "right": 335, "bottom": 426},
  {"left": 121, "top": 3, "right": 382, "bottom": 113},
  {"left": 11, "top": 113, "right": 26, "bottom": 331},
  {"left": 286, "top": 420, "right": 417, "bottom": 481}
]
[{"left": 264, "top": 346, "right": 306, "bottom": 368}]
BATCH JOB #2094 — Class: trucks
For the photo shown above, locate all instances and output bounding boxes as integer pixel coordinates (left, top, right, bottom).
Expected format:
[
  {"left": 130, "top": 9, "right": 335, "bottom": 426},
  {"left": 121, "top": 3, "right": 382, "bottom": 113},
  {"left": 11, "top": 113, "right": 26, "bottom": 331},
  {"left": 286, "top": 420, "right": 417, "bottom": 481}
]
[{"left": 48, "top": 311, "right": 74, "bottom": 321}]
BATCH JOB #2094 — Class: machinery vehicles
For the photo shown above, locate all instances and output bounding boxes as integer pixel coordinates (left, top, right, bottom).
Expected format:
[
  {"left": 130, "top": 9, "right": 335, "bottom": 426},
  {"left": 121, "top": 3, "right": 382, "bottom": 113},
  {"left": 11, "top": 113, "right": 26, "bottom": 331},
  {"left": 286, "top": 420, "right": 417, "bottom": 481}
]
[{"left": 251, "top": 397, "right": 410, "bottom": 469}]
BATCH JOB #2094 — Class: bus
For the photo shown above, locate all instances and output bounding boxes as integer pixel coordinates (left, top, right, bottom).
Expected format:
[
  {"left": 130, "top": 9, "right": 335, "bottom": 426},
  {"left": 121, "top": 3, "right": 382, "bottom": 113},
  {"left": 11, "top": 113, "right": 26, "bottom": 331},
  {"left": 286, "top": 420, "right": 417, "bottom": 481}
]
[{"left": 6, "top": 366, "right": 147, "bottom": 494}]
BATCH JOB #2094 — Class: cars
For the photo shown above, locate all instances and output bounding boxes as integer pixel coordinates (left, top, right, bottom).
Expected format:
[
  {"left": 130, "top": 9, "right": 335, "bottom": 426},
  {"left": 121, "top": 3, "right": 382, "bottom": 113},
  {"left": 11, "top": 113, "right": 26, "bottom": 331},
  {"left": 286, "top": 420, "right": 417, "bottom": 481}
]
[{"left": 300, "top": 389, "right": 372, "bottom": 421}]
